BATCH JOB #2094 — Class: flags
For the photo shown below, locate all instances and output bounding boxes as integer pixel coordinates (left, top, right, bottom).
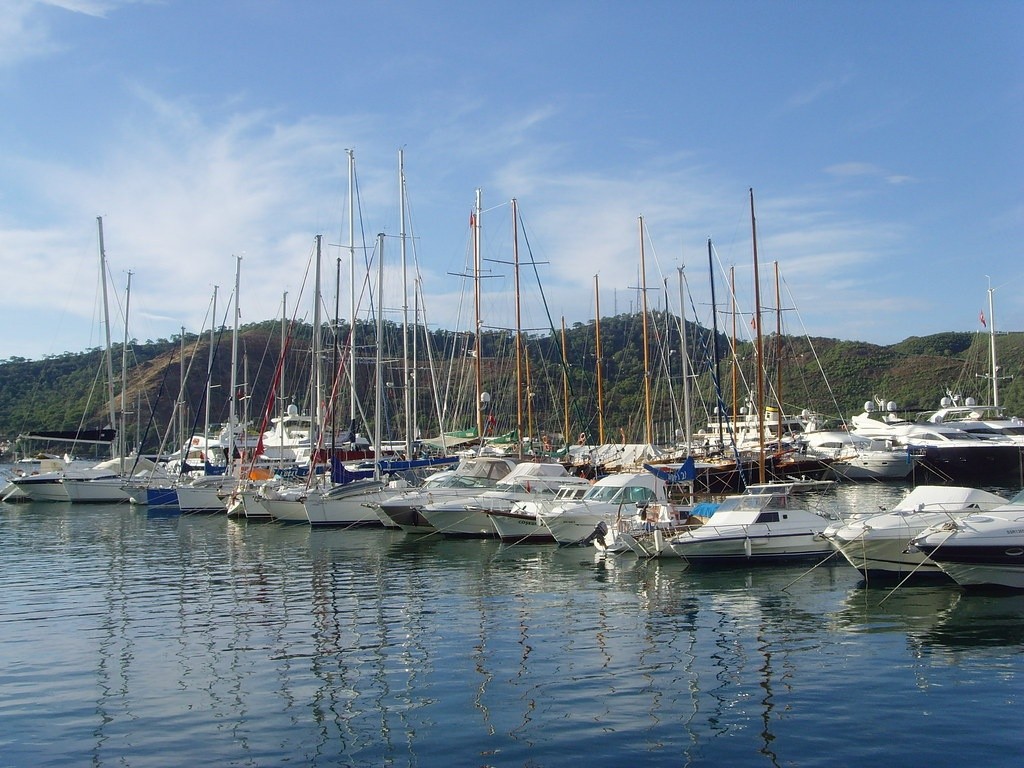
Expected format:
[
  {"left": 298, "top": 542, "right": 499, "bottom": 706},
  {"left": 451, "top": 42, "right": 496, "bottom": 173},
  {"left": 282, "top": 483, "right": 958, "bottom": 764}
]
[
  {"left": 980, "top": 308, "right": 987, "bottom": 326},
  {"left": 469, "top": 210, "right": 474, "bottom": 229},
  {"left": 750, "top": 316, "right": 756, "bottom": 329}
]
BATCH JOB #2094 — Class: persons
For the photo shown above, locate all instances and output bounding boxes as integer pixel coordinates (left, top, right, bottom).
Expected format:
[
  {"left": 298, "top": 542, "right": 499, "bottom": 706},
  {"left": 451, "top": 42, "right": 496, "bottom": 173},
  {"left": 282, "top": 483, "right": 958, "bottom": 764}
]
[
  {"left": 413, "top": 452, "right": 417, "bottom": 461},
  {"left": 199, "top": 452, "right": 204, "bottom": 462}
]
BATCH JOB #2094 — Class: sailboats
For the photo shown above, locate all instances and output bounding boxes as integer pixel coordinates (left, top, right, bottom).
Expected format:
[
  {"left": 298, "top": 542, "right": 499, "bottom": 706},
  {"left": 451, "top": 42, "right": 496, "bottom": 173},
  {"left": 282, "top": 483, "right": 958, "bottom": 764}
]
[{"left": 1, "top": 146, "right": 1023, "bottom": 598}]
